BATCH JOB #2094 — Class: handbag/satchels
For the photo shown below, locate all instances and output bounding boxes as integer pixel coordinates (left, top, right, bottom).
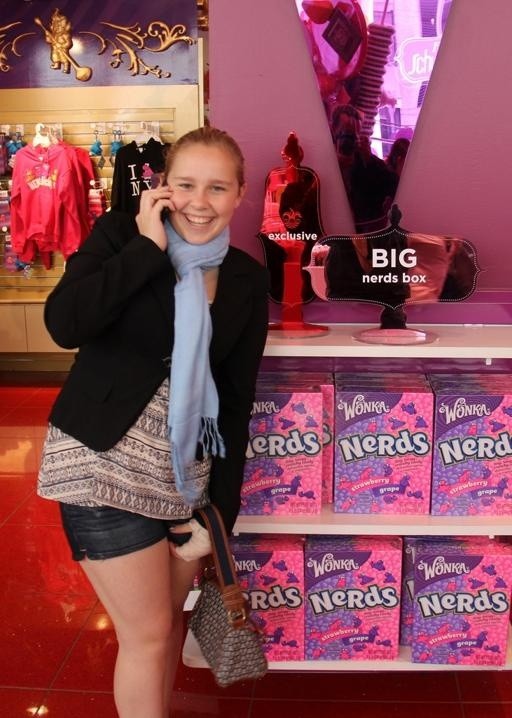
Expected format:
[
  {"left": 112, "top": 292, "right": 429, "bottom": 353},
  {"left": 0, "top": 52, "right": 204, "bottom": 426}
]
[{"left": 184, "top": 570, "right": 269, "bottom": 698}]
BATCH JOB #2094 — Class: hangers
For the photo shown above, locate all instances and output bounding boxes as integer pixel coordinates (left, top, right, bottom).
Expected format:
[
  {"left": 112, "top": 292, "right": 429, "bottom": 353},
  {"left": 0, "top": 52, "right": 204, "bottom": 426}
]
[
  {"left": 29, "top": 123, "right": 61, "bottom": 149},
  {"left": 133, "top": 121, "right": 165, "bottom": 146}
]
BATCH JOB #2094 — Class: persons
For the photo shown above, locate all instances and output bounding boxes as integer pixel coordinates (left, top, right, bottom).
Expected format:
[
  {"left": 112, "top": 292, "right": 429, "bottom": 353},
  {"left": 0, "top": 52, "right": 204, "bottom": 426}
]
[
  {"left": 331, "top": 104, "right": 410, "bottom": 232},
  {"left": 35, "top": 126, "right": 269, "bottom": 717}
]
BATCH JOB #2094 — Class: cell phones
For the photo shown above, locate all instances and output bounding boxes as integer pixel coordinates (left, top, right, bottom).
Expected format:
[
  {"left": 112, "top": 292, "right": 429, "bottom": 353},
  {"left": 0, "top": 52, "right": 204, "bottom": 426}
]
[{"left": 151, "top": 174, "right": 169, "bottom": 224}]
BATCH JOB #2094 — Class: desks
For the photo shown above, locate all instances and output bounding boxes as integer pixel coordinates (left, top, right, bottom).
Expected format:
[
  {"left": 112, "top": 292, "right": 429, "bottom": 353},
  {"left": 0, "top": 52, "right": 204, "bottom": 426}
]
[{"left": 0, "top": 287, "right": 79, "bottom": 352}]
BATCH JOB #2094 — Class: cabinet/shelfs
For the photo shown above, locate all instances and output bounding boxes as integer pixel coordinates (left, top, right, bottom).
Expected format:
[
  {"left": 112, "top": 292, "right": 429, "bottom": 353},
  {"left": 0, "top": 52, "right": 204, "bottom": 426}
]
[{"left": 181, "top": 324, "right": 512, "bottom": 671}]
